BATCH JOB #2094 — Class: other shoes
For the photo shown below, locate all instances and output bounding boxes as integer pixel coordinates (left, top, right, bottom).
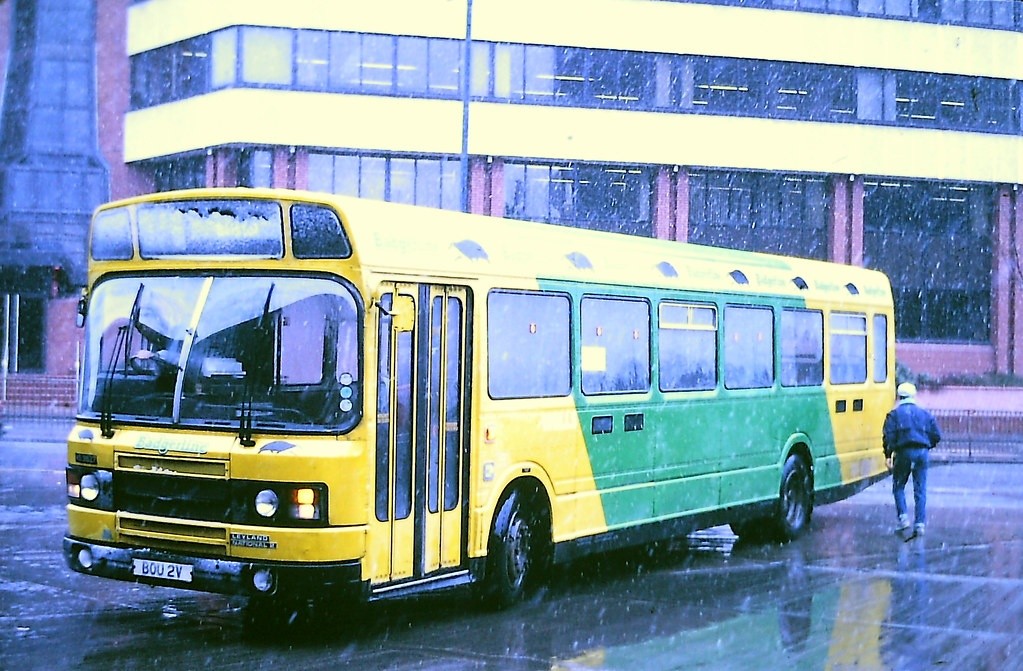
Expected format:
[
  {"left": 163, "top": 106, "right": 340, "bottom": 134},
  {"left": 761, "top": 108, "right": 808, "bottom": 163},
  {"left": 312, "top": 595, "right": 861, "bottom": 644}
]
[
  {"left": 895, "top": 520, "right": 911, "bottom": 531},
  {"left": 913, "top": 528, "right": 924, "bottom": 537}
]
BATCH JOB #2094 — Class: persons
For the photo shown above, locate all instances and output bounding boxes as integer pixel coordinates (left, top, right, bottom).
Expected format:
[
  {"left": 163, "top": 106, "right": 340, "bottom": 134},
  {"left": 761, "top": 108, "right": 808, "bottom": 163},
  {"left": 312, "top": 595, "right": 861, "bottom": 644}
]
[{"left": 882, "top": 381, "right": 941, "bottom": 536}]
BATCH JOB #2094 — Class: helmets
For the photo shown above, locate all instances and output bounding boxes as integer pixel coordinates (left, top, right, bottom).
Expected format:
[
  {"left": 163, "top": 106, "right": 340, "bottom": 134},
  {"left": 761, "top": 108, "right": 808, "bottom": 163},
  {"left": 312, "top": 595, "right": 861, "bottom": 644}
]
[{"left": 898, "top": 383, "right": 917, "bottom": 397}]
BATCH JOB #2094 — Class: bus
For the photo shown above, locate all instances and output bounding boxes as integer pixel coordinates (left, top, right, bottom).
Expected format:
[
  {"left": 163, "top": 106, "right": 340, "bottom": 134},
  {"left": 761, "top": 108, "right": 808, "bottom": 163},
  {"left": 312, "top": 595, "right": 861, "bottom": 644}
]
[{"left": 62, "top": 186, "right": 897, "bottom": 608}]
